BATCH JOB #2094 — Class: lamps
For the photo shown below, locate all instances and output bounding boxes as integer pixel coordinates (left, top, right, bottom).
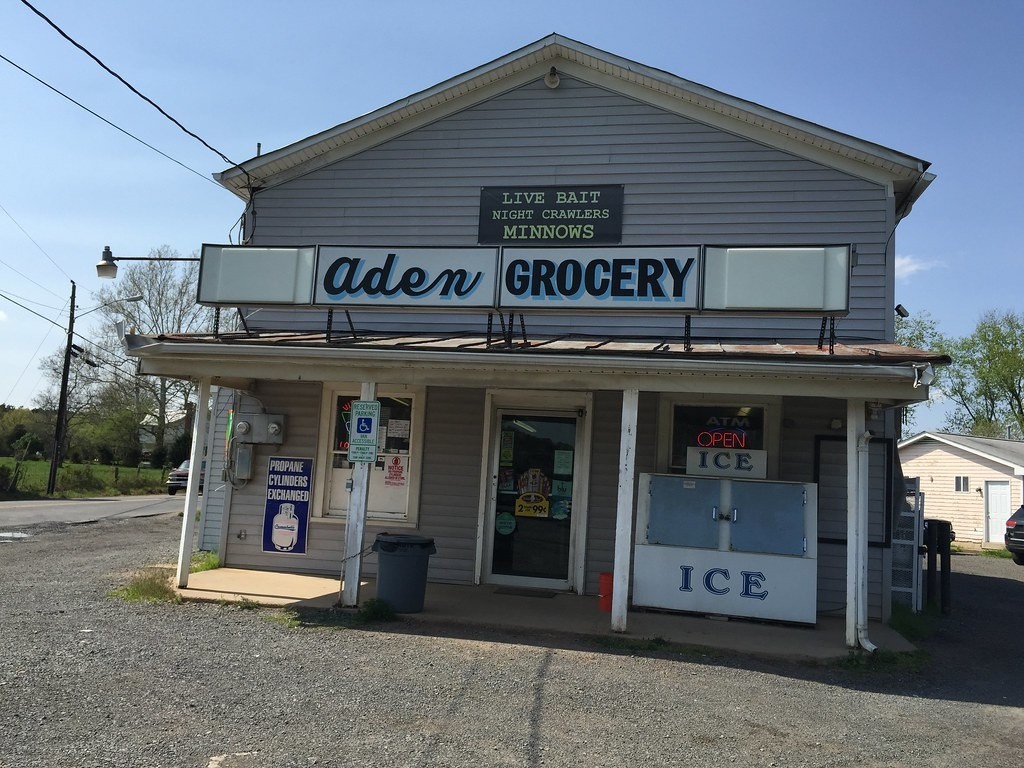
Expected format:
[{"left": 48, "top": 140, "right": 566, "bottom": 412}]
[{"left": 96, "top": 245, "right": 201, "bottom": 279}]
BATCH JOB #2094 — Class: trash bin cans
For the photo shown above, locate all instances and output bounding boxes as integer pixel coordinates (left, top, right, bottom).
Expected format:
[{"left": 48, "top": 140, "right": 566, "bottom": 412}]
[
  {"left": 373, "top": 533, "right": 435, "bottom": 615},
  {"left": 924, "top": 518, "right": 952, "bottom": 553}
]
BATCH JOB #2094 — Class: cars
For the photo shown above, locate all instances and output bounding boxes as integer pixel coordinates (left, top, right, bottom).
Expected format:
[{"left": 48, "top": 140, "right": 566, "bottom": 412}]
[
  {"left": 1004, "top": 504, "right": 1024, "bottom": 566},
  {"left": 165, "top": 459, "right": 206, "bottom": 496}
]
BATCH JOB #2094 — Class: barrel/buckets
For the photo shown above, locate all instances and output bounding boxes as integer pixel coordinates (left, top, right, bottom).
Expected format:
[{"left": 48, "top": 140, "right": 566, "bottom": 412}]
[{"left": 596, "top": 572, "right": 614, "bottom": 613}]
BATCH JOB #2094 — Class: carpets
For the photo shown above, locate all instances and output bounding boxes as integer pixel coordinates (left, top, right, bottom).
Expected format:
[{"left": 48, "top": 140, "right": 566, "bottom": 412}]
[{"left": 494, "top": 585, "right": 557, "bottom": 599}]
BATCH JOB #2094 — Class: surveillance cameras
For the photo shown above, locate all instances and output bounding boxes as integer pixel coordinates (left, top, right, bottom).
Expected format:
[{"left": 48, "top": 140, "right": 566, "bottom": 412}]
[{"left": 895, "top": 304, "right": 909, "bottom": 318}]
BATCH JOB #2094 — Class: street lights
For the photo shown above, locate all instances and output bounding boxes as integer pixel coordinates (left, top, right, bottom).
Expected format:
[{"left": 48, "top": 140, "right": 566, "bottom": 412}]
[{"left": 42, "top": 283, "right": 144, "bottom": 497}]
